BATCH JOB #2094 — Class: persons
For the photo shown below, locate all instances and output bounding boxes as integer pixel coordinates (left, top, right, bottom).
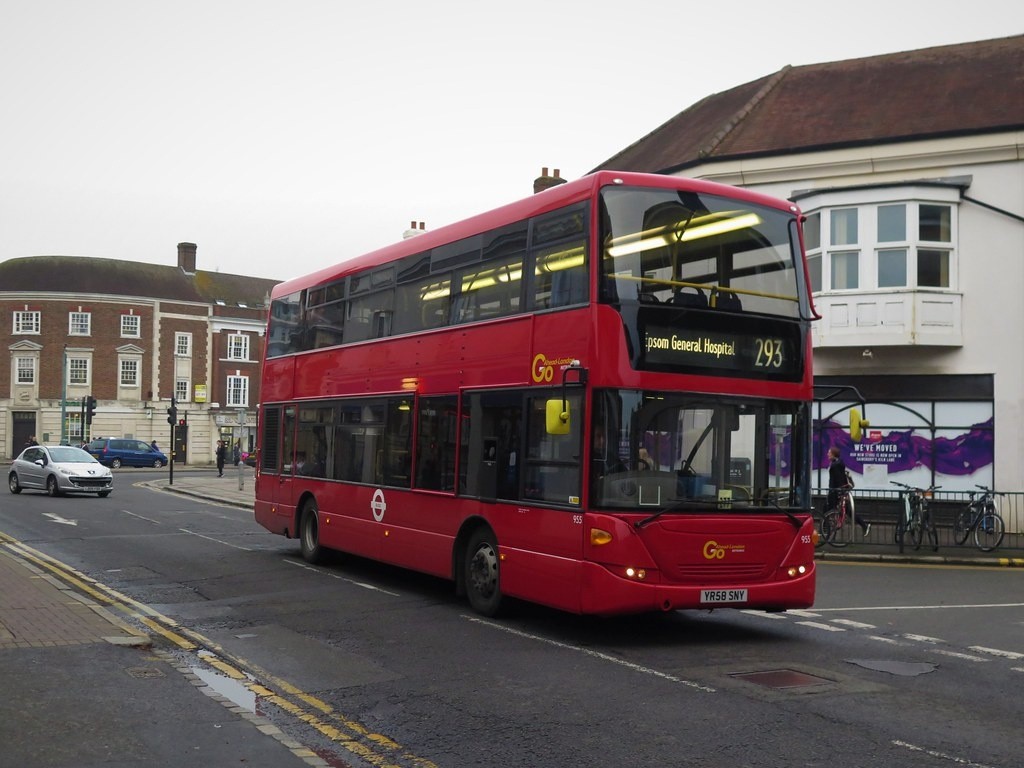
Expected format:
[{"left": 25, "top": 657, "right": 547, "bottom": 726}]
[
  {"left": 151, "top": 440, "right": 160, "bottom": 451},
  {"left": 592, "top": 423, "right": 653, "bottom": 485},
  {"left": 81, "top": 440, "right": 89, "bottom": 452},
  {"left": 817, "top": 447, "right": 871, "bottom": 537},
  {"left": 30, "top": 436, "right": 40, "bottom": 446},
  {"left": 215, "top": 440, "right": 226, "bottom": 478},
  {"left": 234, "top": 441, "right": 240, "bottom": 466}
]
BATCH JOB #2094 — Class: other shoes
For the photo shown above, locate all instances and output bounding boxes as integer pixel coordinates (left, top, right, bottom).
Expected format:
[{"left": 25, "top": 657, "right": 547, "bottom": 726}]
[
  {"left": 217, "top": 474, "right": 223, "bottom": 477},
  {"left": 863, "top": 523, "right": 871, "bottom": 536}
]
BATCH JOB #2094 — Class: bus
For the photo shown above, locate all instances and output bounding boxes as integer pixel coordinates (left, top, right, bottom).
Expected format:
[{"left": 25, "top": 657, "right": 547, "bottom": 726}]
[{"left": 252, "top": 169, "right": 871, "bottom": 619}]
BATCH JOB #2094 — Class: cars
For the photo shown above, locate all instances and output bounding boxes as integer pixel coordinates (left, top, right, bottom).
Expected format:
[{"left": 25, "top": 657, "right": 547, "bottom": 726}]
[
  {"left": 7, "top": 445, "right": 114, "bottom": 498},
  {"left": 240, "top": 451, "right": 257, "bottom": 467},
  {"left": 81, "top": 438, "right": 168, "bottom": 469}
]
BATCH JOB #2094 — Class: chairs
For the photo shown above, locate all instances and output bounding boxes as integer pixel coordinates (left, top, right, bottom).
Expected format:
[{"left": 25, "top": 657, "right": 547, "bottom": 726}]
[{"left": 663, "top": 280, "right": 744, "bottom": 311}]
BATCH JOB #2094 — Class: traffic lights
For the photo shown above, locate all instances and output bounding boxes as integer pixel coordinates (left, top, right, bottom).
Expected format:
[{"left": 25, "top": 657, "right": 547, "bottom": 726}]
[
  {"left": 179, "top": 419, "right": 185, "bottom": 426},
  {"left": 166, "top": 406, "right": 177, "bottom": 426},
  {"left": 86, "top": 395, "right": 97, "bottom": 424}
]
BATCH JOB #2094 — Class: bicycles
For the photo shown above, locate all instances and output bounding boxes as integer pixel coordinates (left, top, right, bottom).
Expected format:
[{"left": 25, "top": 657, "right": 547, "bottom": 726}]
[
  {"left": 889, "top": 480, "right": 943, "bottom": 554},
  {"left": 769, "top": 493, "right": 830, "bottom": 549},
  {"left": 951, "top": 484, "right": 1006, "bottom": 553},
  {"left": 820, "top": 483, "right": 856, "bottom": 548}
]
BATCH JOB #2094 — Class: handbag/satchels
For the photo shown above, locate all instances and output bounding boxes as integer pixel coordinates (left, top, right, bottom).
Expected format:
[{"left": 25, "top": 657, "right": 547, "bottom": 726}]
[{"left": 844, "top": 470, "right": 854, "bottom": 489}]
